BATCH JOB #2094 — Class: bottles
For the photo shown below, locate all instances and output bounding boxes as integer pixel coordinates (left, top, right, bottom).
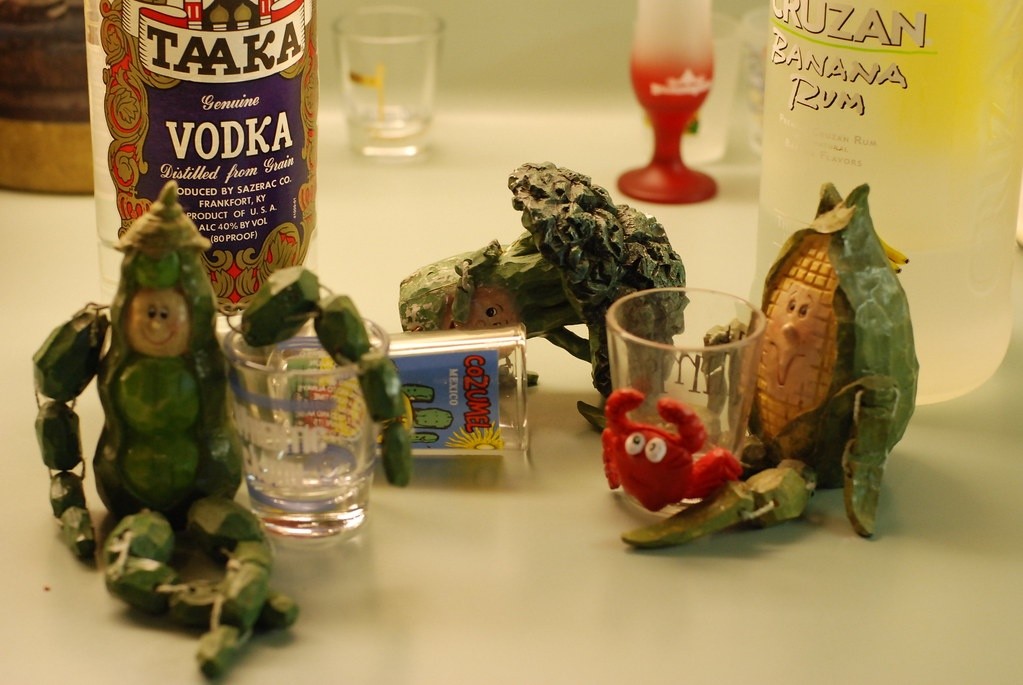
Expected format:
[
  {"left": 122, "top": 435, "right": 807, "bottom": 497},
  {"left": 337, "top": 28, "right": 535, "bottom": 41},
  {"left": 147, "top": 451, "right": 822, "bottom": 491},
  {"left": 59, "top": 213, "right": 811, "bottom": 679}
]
[
  {"left": 757, "top": 0, "right": 1023, "bottom": 405},
  {"left": 83, "top": 0, "right": 320, "bottom": 334}
]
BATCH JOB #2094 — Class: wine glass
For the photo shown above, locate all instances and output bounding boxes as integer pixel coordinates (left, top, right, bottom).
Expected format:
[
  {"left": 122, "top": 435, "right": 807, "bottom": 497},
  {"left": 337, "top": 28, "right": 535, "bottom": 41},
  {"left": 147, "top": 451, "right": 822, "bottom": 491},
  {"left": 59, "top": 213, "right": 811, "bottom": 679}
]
[{"left": 619, "top": 0, "right": 718, "bottom": 203}]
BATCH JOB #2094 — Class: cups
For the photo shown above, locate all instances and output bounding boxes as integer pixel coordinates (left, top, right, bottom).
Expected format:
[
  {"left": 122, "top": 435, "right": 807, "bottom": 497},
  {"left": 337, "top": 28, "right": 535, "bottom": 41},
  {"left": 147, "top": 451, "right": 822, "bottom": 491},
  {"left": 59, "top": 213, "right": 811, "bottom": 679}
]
[
  {"left": 223, "top": 312, "right": 387, "bottom": 541},
  {"left": 365, "top": 324, "right": 531, "bottom": 456},
  {"left": 336, "top": 10, "right": 444, "bottom": 160},
  {"left": 606, "top": 286, "right": 765, "bottom": 514}
]
